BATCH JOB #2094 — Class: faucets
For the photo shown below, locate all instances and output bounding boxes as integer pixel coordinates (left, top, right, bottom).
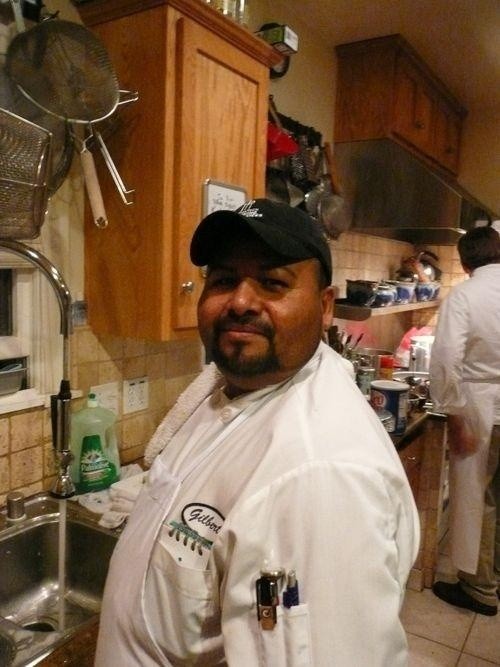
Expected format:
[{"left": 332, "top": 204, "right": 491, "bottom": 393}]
[{"left": 1, "top": 234, "right": 73, "bottom": 338}]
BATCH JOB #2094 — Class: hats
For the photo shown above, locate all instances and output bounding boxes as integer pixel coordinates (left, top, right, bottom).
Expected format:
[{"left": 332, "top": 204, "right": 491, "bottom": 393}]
[{"left": 189, "top": 196, "right": 333, "bottom": 287}]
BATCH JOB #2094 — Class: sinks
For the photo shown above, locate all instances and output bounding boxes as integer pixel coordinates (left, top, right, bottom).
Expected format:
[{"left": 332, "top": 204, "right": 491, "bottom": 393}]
[
  {"left": 0, "top": 490, "right": 126, "bottom": 631},
  {"left": 1, "top": 632, "right": 18, "bottom": 667}
]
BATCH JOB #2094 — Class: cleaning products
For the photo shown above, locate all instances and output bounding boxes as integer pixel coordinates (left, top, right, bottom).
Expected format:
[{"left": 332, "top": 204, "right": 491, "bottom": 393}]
[{"left": 67, "top": 393, "right": 121, "bottom": 495}]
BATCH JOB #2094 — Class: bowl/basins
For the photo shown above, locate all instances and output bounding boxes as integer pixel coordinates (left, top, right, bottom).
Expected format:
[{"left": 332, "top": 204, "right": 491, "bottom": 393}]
[
  {"left": 393, "top": 371, "right": 430, "bottom": 384},
  {"left": 346, "top": 279, "right": 436, "bottom": 308},
  {"left": 0, "top": 367, "right": 28, "bottom": 395}
]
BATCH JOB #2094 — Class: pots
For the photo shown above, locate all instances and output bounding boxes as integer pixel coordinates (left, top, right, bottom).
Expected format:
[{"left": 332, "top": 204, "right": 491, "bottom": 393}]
[{"left": 333, "top": 139, "right": 500, "bottom": 248}]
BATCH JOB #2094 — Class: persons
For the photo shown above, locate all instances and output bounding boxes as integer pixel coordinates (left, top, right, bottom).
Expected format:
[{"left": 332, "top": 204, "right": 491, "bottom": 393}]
[
  {"left": 92, "top": 200, "right": 419, "bottom": 667},
  {"left": 426, "top": 225, "right": 500, "bottom": 616}
]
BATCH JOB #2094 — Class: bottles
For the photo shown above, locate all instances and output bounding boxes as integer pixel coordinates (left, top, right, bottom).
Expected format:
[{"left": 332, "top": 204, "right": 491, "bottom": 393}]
[{"left": 351, "top": 348, "right": 394, "bottom": 380}]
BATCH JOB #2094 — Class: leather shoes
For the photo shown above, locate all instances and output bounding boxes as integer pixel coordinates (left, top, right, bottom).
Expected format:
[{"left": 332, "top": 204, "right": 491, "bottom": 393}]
[{"left": 432, "top": 579, "right": 497, "bottom": 616}]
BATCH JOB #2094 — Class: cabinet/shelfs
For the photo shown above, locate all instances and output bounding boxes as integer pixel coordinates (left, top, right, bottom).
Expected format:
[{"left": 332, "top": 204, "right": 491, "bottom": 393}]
[
  {"left": 82, "top": 0, "right": 286, "bottom": 350},
  {"left": 337, "top": 34, "right": 468, "bottom": 183}
]
